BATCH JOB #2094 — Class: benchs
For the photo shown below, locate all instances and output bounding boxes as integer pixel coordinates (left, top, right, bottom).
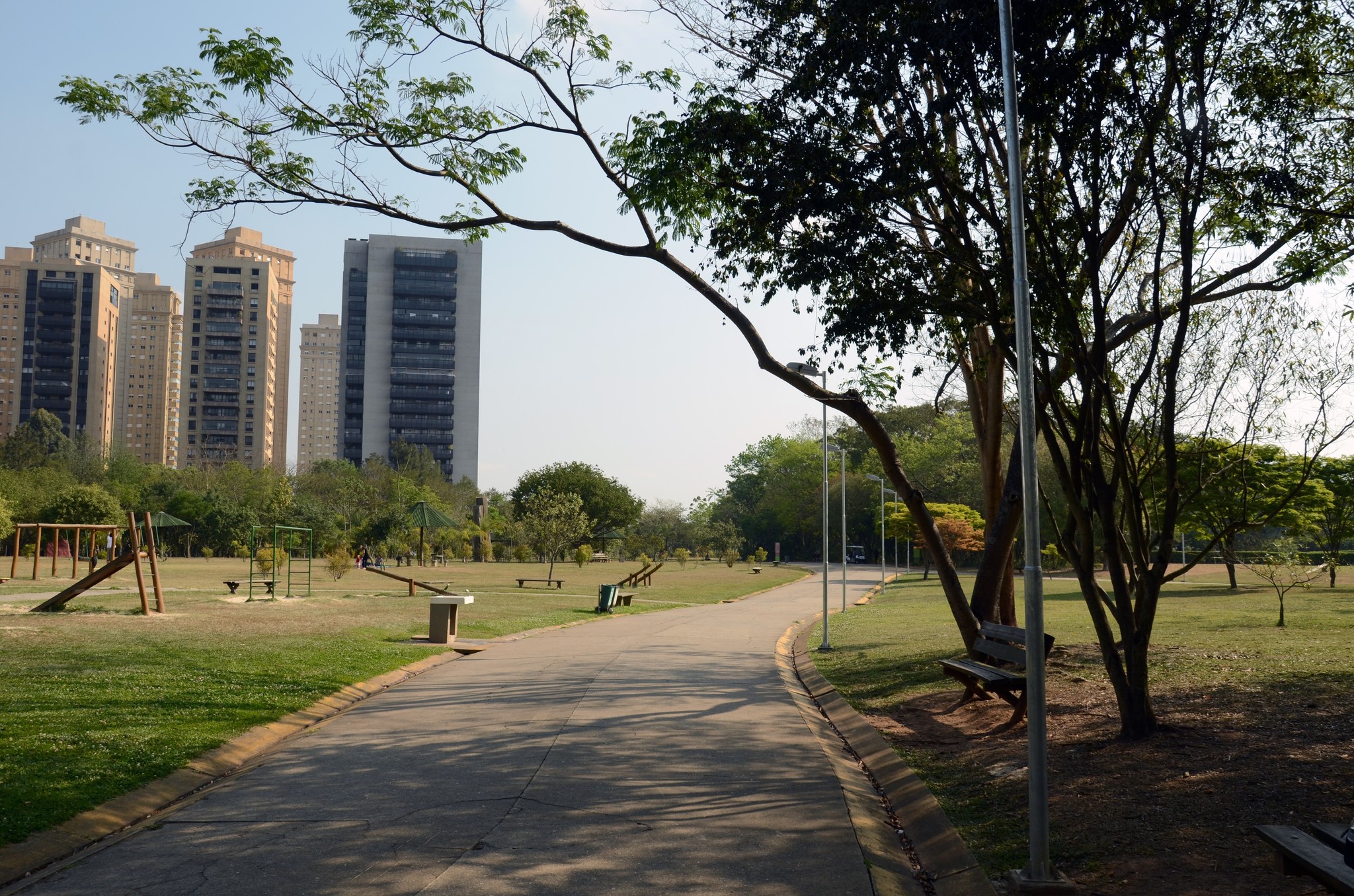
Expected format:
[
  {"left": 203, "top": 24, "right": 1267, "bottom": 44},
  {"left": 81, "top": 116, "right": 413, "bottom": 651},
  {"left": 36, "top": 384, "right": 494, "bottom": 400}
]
[
  {"left": 223, "top": 580, "right": 281, "bottom": 594},
  {"left": 615, "top": 593, "right": 639, "bottom": 607},
  {"left": 515, "top": 579, "right": 565, "bottom": 590},
  {"left": 591, "top": 553, "right": 609, "bottom": 562},
  {"left": 773, "top": 561, "right": 779, "bottom": 567},
  {"left": 938, "top": 620, "right": 1055, "bottom": 738},
  {"left": 753, "top": 567, "right": 762, "bottom": 574},
  {"left": 1250, "top": 817, "right": 1354, "bottom": 896}
]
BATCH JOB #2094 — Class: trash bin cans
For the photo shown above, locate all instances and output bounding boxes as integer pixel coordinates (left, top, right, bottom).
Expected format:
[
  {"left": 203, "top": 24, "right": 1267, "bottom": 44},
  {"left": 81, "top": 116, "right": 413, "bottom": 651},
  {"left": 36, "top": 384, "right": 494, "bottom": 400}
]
[
  {"left": 610, "top": 584, "right": 620, "bottom": 608},
  {"left": 600, "top": 585, "right": 615, "bottom": 609}
]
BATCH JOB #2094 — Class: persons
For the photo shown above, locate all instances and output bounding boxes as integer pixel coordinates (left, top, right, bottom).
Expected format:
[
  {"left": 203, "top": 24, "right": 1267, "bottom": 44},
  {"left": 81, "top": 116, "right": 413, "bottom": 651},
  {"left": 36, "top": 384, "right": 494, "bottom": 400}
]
[
  {"left": 814, "top": 549, "right": 820, "bottom": 562},
  {"left": 106, "top": 528, "right": 119, "bottom": 564},
  {"left": 355, "top": 554, "right": 361, "bottom": 569},
  {"left": 872, "top": 548, "right": 879, "bottom": 564},
  {"left": 358, "top": 544, "right": 369, "bottom": 569},
  {"left": 852, "top": 548, "right": 856, "bottom": 558},
  {"left": 405, "top": 550, "right": 414, "bottom": 556},
  {"left": 374, "top": 554, "right": 383, "bottom": 567}
]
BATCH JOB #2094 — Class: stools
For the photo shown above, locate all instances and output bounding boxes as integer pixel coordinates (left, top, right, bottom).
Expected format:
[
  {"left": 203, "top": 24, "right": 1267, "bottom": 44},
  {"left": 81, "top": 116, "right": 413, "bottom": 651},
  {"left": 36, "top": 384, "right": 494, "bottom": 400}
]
[
  {"left": 431, "top": 560, "right": 449, "bottom": 567},
  {"left": 417, "top": 559, "right": 427, "bottom": 566}
]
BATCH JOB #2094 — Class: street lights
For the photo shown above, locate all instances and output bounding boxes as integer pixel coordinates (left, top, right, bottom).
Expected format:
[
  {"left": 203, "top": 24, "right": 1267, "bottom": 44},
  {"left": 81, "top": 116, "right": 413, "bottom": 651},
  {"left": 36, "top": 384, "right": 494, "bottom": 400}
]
[
  {"left": 786, "top": 361, "right": 832, "bottom": 651},
  {"left": 897, "top": 497, "right": 910, "bottom": 575},
  {"left": 819, "top": 443, "right": 846, "bottom": 613},
  {"left": 866, "top": 474, "right": 885, "bottom": 595},
  {"left": 883, "top": 488, "right": 898, "bottom": 582}
]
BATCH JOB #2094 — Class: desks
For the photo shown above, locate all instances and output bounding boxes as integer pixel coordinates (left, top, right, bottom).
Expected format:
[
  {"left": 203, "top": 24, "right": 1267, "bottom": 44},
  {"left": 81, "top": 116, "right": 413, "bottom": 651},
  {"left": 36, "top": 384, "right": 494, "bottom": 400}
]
[
  {"left": 429, "top": 555, "right": 443, "bottom": 566},
  {"left": 401, "top": 555, "right": 414, "bottom": 566}
]
